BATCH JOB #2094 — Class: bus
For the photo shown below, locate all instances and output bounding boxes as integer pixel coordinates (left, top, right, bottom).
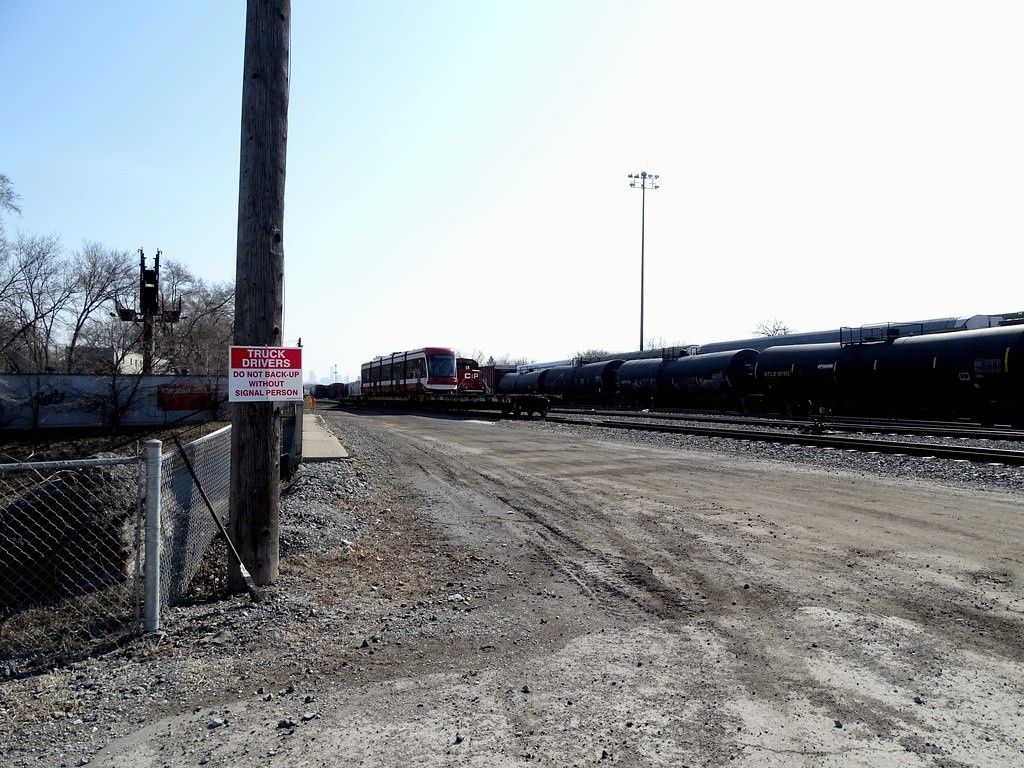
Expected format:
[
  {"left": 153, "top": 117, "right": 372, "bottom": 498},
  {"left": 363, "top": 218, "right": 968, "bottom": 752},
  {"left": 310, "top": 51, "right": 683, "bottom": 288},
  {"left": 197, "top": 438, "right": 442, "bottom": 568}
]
[
  {"left": 360, "top": 348, "right": 460, "bottom": 397},
  {"left": 455, "top": 358, "right": 488, "bottom": 396}
]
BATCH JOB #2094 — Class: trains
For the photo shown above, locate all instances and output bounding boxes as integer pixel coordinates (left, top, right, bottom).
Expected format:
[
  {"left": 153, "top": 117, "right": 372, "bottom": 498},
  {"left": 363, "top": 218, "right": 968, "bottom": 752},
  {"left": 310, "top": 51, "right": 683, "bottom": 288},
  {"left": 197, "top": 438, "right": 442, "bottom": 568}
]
[
  {"left": 309, "top": 381, "right": 361, "bottom": 400},
  {"left": 489, "top": 312, "right": 1024, "bottom": 429}
]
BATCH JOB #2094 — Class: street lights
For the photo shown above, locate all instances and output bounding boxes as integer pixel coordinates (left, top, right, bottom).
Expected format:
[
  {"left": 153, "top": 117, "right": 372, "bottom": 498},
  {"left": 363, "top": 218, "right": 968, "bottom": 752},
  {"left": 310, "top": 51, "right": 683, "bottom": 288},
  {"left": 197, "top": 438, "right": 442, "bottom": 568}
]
[
  {"left": 629, "top": 172, "right": 660, "bottom": 353},
  {"left": 334, "top": 364, "right": 338, "bottom": 381}
]
